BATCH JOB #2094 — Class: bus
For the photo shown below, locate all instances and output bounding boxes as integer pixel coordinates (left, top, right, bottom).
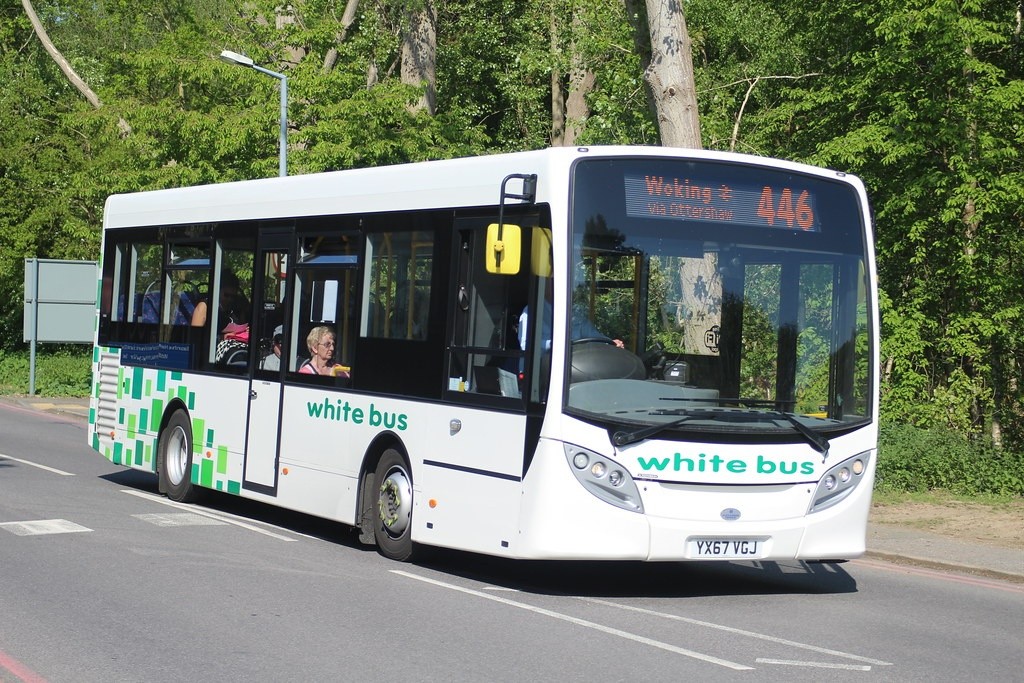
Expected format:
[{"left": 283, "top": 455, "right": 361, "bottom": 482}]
[{"left": 87, "top": 144, "right": 882, "bottom": 565}]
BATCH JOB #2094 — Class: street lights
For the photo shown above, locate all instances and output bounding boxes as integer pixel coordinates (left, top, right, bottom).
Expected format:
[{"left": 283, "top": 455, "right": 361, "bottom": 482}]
[{"left": 219, "top": 49, "right": 288, "bottom": 178}]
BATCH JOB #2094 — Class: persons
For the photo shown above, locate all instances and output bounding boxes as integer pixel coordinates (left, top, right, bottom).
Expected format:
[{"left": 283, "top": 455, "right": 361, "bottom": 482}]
[
  {"left": 192, "top": 268, "right": 351, "bottom": 377},
  {"left": 518, "top": 276, "right": 625, "bottom": 373}
]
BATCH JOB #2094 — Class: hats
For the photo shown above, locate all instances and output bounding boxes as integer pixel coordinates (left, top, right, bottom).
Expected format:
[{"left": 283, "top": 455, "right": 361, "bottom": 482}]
[{"left": 271, "top": 325, "right": 282, "bottom": 340}]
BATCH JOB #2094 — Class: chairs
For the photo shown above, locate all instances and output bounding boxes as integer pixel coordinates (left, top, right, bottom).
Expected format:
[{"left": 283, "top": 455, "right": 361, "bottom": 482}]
[
  {"left": 120, "top": 279, "right": 396, "bottom": 371},
  {"left": 485, "top": 305, "right": 521, "bottom": 369}
]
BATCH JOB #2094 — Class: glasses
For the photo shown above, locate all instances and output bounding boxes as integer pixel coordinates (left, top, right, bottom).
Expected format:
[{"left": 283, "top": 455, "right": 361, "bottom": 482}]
[
  {"left": 222, "top": 289, "right": 236, "bottom": 300},
  {"left": 318, "top": 342, "right": 336, "bottom": 348},
  {"left": 275, "top": 338, "right": 282, "bottom": 343}
]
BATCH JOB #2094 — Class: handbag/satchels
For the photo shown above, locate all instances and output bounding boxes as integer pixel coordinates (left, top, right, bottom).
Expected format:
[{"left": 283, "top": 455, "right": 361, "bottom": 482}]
[{"left": 225, "top": 331, "right": 249, "bottom": 343}]
